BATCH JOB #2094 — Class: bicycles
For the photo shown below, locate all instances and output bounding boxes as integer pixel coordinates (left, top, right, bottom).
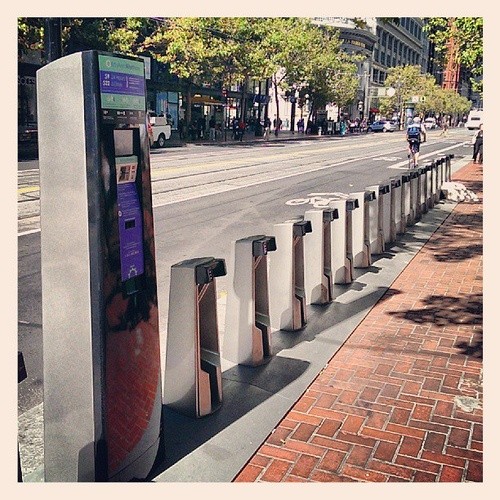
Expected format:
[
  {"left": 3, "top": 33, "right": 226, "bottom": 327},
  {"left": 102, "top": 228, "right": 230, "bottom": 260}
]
[{"left": 408, "top": 138, "right": 424, "bottom": 170}]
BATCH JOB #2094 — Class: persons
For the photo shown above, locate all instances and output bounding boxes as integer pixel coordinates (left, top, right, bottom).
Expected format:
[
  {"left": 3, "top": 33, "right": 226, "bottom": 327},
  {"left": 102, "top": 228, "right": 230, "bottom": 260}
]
[
  {"left": 155, "top": 111, "right": 375, "bottom": 143},
  {"left": 472, "top": 128, "right": 483, "bottom": 164},
  {"left": 406, "top": 117, "right": 427, "bottom": 168}
]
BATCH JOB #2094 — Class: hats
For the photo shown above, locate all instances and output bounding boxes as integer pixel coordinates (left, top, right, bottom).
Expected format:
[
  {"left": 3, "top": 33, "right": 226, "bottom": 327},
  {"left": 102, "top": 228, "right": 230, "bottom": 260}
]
[{"left": 413, "top": 117, "right": 421, "bottom": 123}]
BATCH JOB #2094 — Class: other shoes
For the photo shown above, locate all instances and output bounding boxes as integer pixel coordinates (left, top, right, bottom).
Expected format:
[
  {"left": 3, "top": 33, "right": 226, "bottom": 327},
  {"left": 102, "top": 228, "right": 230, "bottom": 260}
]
[
  {"left": 414, "top": 164, "right": 418, "bottom": 168},
  {"left": 473, "top": 160, "right": 476, "bottom": 164},
  {"left": 408, "top": 154, "right": 411, "bottom": 158},
  {"left": 478, "top": 160, "right": 481, "bottom": 164}
]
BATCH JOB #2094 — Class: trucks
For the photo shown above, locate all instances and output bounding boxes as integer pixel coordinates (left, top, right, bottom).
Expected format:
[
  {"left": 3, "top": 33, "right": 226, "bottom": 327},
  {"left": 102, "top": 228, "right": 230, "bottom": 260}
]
[{"left": 464, "top": 110, "right": 483, "bottom": 130}]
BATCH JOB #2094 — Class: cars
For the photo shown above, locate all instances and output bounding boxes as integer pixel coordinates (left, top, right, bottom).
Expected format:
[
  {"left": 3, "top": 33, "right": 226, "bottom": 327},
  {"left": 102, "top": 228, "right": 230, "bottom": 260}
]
[
  {"left": 423, "top": 117, "right": 437, "bottom": 129},
  {"left": 367, "top": 119, "right": 396, "bottom": 132}
]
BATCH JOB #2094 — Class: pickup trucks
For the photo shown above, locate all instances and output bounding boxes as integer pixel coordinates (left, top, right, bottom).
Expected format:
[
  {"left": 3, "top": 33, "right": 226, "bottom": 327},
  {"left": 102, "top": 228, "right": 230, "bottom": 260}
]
[{"left": 150, "top": 125, "right": 173, "bottom": 148}]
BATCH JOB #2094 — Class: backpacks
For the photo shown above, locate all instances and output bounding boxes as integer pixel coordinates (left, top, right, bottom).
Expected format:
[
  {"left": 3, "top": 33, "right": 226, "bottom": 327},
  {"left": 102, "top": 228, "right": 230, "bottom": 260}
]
[{"left": 407, "top": 123, "right": 421, "bottom": 144}]
